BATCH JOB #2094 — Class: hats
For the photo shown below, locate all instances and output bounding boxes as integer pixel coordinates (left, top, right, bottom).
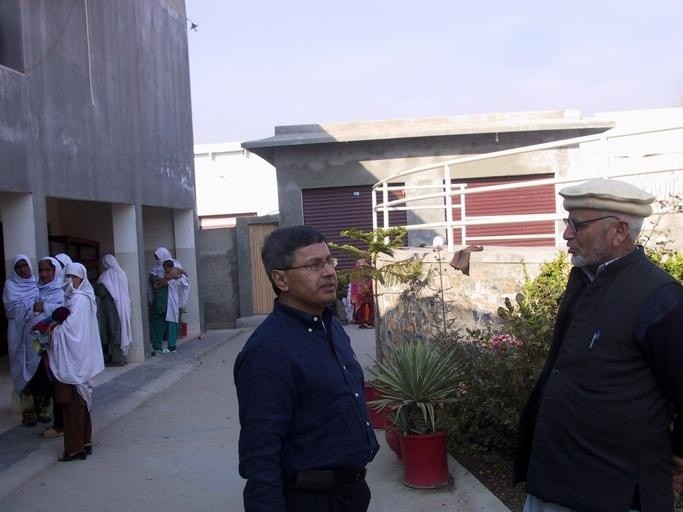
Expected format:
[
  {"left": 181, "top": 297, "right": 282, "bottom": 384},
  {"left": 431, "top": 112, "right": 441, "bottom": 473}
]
[{"left": 557, "top": 179, "right": 656, "bottom": 217}]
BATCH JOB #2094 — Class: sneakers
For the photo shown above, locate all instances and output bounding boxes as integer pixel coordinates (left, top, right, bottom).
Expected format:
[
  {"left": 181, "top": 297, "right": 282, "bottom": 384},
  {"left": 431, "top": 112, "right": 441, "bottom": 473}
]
[
  {"left": 22, "top": 408, "right": 38, "bottom": 426},
  {"left": 35, "top": 406, "right": 52, "bottom": 424},
  {"left": 45, "top": 427, "right": 54, "bottom": 431},
  {"left": 359, "top": 323, "right": 372, "bottom": 329},
  {"left": 38, "top": 429, "right": 64, "bottom": 439},
  {"left": 103, "top": 356, "right": 127, "bottom": 366}
]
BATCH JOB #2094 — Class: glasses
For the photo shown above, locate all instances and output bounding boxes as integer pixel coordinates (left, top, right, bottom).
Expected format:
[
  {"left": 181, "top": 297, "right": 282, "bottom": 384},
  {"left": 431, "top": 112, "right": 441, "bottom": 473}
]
[
  {"left": 276, "top": 257, "right": 338, "bottom": 271},
  {"left": 562, "top": 216, "right": 620, "bottom": 234}
]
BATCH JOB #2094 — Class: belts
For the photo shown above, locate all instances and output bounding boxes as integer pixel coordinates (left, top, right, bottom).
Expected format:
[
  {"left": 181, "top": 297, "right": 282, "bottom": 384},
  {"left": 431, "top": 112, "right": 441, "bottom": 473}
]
[{"left": 283, "top": 468, "right": 367, "bottom": 489}]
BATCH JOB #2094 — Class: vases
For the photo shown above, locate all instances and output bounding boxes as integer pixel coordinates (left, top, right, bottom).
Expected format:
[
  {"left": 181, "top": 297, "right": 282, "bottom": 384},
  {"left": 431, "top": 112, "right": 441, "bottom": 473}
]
[
  {"left": 381, "top": 418, "right": 402, "bottom": 456},
  {"left": 363, "top": 377, "right": 393, "bottom": 432}
]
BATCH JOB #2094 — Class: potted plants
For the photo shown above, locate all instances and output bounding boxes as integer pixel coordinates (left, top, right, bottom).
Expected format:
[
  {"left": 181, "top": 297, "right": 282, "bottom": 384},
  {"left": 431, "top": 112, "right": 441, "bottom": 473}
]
[{"left": 363, "top": 337, "right": 472, "bottom": 489}]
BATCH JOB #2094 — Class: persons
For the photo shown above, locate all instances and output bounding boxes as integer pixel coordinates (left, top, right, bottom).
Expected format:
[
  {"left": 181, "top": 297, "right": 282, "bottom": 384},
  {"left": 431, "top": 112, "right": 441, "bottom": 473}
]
[
  {"left": 94, "top": 255, "right": 133, "bottom": 367},
  {"left": 515, "top": 176, "right": 683, "bottom": 511},
  {"left": 147, "top": 247, "right": 188, "bottom": 354},
  {"left": 230, "top": 224, "right": 380, "bottom": 510},
  {"left": 346, "top": 257, "right": 374, "bottom": 329},
  {"left": 2, "top": 253, "right": 106, "bottom": 462}
]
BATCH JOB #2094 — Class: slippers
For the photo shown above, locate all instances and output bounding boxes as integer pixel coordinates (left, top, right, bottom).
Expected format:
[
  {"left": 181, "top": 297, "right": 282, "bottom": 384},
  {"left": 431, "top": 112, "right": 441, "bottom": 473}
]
[
  {"left": 150, "top": 348, "right": 165, "bottom": 354},
  {"left": 163, "top": 347, "right": 177, "bottom": 353}
]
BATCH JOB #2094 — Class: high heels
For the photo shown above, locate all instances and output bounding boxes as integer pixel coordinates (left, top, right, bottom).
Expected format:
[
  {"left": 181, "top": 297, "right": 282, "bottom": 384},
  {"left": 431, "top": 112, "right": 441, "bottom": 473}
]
[
  {"left": 83, "top": 445, "right": 93, "bottom": 455},
  {"left": 57, "top": 449, "right": 86, "bottom": 461}
]
